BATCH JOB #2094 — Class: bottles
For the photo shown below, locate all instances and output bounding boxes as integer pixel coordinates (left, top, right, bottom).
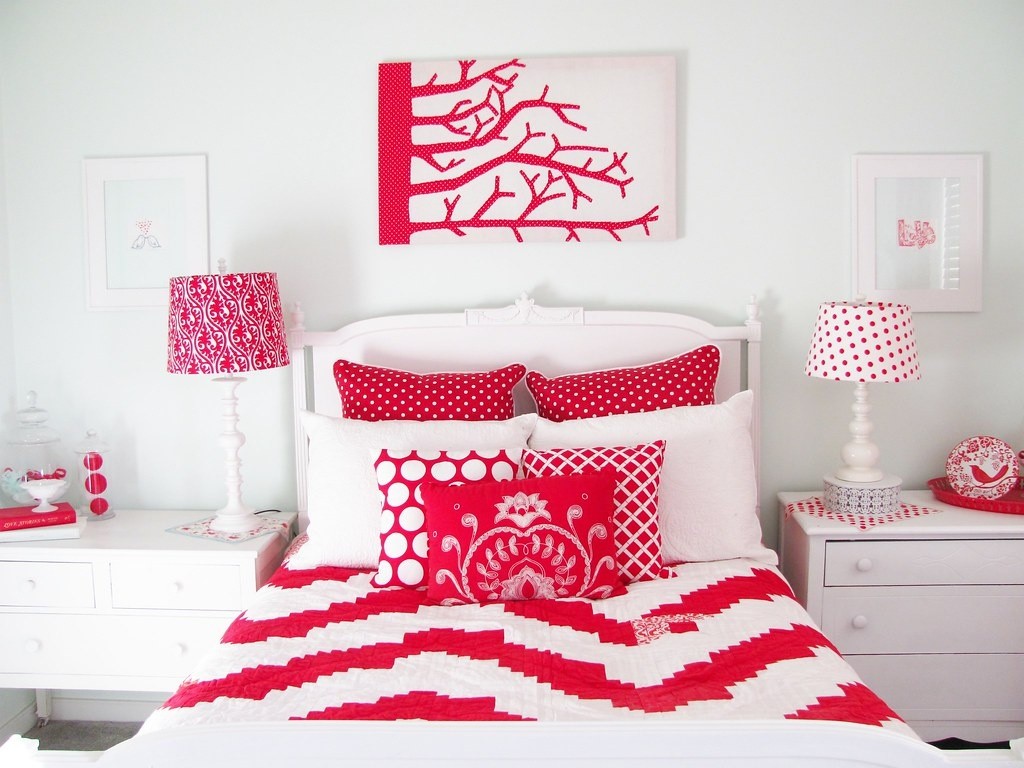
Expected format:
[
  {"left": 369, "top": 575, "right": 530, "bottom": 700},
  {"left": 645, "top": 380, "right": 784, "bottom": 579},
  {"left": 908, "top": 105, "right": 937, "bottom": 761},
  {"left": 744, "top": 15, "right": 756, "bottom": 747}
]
[{"left": 8, "top": 391, "right": 62, "bottom": 491}]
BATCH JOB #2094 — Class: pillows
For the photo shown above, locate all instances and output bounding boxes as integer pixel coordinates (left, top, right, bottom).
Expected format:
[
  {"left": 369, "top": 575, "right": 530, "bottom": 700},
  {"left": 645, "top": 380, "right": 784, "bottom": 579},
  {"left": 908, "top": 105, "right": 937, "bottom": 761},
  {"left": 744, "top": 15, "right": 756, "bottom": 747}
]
[{"left": 298, "top": 345, "right": 763, "bottom": 601}]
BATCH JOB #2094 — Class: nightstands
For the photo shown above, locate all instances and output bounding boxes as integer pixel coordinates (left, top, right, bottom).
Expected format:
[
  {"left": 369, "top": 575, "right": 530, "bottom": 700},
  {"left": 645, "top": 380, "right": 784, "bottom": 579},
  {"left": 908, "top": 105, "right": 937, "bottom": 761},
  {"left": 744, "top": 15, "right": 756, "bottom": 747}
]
[
  {"left": 778, "top": 491, "right": 1024, "bottom": 750},
  {"left": 0, "top": 510, "right": 300, "bottom": 722}
]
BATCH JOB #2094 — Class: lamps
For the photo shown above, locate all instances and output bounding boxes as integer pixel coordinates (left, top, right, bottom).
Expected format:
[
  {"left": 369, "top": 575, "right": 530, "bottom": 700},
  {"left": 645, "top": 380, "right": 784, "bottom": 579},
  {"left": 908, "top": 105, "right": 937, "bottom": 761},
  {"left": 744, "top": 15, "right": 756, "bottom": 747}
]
[
  {"left": 168, "top": 271, "right": 291, "bottom": 534},
  {"left": 803, "top": 301, "right": 922, "bottom": 516}
]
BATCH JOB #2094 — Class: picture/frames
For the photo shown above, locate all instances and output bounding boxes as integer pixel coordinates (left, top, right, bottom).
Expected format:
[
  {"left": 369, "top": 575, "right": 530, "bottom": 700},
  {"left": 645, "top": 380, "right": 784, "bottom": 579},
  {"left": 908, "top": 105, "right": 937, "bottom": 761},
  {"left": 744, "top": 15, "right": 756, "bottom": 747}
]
[
  {"left": 377, "top": 55, "right": 676, "bottom": 246},
  {"left": 851, "top": 152, "right": 983, "bottom": 312},
  {"left": 82, "top": 155, "right": 211, "bottom": 311}
]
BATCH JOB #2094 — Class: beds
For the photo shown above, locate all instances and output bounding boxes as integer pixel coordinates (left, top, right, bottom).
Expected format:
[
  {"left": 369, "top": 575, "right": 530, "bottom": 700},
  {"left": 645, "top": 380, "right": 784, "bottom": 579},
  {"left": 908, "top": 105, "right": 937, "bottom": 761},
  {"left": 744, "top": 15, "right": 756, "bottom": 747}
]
[{"left": 94, "top": 291, "right": 950, "bottom": 766}]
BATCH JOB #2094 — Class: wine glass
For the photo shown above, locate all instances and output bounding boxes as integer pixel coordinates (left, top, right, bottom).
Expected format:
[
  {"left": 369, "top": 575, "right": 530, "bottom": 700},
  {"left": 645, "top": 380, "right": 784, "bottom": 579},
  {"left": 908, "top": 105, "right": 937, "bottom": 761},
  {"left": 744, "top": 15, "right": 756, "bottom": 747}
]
[{"left": 20, "top": 479, "right": 68, "bottom": 513}]
